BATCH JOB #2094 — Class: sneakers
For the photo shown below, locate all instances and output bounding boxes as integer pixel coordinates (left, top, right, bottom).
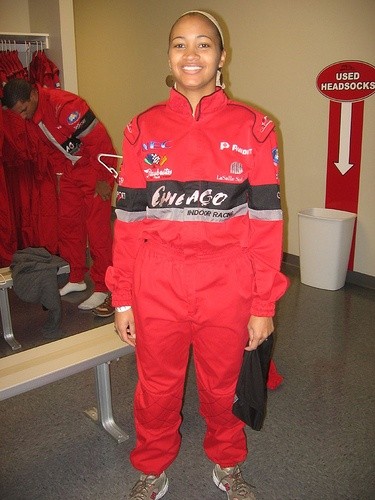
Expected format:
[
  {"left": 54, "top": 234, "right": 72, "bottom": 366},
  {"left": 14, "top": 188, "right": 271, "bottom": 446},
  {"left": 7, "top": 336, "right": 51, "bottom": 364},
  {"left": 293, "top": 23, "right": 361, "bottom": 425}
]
[
  {"left": 212, "top": 464, "right": 256, "bottom": 500},
  {"left": 126, "top": 470, "right": 169, "bottom": 500},
  {"left": 92, "top": 292, "right": 115, "bottom": 317}
]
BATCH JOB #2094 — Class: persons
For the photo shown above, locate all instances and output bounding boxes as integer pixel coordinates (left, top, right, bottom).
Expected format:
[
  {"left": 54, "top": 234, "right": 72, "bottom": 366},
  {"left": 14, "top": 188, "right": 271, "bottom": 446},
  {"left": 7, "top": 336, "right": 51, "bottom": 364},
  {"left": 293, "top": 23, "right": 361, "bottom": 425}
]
[
  {"left": 104, "top": 9, "right": 290, "bottom": 500},
  {"left": 4, "top": 78, "right": 119, "bottom": 310}
]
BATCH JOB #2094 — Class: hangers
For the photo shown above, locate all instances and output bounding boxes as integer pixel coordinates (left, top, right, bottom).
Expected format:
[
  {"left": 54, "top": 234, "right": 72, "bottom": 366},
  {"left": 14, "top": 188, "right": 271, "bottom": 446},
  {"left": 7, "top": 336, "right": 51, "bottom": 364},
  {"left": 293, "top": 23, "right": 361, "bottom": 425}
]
[{"left": 1, "top": 40, "right": 44, "bottom": 78}]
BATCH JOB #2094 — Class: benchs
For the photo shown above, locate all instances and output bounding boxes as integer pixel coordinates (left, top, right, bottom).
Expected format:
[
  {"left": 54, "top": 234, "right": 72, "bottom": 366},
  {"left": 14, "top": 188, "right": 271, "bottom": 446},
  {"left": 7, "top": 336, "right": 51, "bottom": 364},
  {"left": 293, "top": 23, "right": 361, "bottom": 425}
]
[
  {"left": 0, "top": 290, "right": 137, "bottom": 444},
  {"left": 0, "top": 254, "right": 70, "bottom": 351}
]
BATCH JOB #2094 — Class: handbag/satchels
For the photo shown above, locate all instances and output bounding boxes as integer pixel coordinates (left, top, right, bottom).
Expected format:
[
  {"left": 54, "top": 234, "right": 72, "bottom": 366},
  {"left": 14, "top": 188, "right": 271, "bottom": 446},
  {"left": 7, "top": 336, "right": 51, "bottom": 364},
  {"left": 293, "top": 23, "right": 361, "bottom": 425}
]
[{"left": 232, "top": 333, "right": 274, "bottom": 432}]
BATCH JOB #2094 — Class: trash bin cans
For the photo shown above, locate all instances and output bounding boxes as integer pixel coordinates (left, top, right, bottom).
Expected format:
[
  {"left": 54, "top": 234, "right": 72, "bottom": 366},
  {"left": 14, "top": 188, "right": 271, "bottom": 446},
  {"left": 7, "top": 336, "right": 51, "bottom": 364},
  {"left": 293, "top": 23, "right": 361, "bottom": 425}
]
[{"left": 297, "top": 208, "right": 356, "bottom": 290}]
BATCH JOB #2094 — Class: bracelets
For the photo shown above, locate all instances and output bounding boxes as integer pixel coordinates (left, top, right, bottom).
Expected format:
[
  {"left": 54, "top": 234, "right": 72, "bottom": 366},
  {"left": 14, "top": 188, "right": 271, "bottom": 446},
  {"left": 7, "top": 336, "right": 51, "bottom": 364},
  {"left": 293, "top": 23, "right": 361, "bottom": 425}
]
[{"left": 115, "top": 306, "right": 131, "bottom": 312}]
